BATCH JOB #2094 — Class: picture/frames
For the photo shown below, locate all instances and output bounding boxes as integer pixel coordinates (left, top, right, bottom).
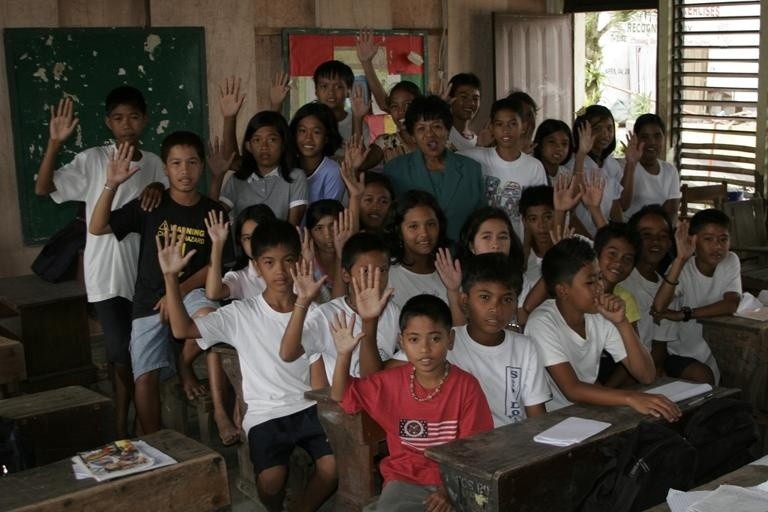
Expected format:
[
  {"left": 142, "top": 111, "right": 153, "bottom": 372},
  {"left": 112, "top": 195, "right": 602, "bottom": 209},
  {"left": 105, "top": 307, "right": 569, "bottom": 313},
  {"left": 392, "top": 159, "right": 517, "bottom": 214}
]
[{"left": 282, "top": 27, "right": 428, "bottom": 124}]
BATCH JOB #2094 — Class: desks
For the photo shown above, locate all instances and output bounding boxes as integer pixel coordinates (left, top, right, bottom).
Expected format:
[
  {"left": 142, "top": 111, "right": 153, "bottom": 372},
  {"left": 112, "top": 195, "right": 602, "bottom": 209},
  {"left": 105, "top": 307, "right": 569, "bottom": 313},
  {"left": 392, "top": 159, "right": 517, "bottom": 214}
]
[
  {"left": 211, "top": 344, "right": 248, "bottom": 495},
  {"left": 305, "top": 386, "right": 386, "bottom": 512},
  {"left": 0, "top": 386, "right": 112, "bottom": 471},
  {"left": 697, "top": 315, "right": 768, "bottom": 444},
  {"left": 642, "top": 455, "right": 768, "bottom": 512},
  {"left": 0, "top": 429, "right": 231, "bottom": 512},
  {"left": 424, "top": 378, "right": 743, "bottom": 512},
  {"left": 0, "top": 336, "right": 27, "bottom": 399}
]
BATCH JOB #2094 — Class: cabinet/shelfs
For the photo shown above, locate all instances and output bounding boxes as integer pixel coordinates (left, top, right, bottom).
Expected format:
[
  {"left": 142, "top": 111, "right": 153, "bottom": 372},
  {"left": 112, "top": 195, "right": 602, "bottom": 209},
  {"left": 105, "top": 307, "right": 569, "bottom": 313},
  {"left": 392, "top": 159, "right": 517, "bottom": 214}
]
[{"left": 0, "top": 275, "right": 104, "bottom": 394}]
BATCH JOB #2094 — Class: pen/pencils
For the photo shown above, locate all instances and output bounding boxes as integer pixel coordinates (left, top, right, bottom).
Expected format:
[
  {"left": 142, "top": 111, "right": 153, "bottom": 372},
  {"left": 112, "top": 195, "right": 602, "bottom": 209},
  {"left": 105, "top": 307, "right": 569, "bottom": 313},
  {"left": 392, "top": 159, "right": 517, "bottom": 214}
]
[{"left": 689, "top": 392, "right": 713, "bottom": 406}]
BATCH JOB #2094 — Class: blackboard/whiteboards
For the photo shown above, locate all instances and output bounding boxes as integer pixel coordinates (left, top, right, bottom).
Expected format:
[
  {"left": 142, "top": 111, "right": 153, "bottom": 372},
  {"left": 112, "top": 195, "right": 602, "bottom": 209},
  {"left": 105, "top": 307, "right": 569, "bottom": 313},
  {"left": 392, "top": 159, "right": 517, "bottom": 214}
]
[{"left": 3, "top": 26, "right": 211, "bottom": 247}]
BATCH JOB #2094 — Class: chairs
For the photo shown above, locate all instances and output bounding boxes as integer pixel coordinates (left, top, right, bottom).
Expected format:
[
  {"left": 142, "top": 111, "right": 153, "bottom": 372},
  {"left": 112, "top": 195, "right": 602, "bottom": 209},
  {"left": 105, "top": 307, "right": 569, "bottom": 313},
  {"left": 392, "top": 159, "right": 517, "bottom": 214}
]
[{"left": 716, "top": 200, "right": 768, "bottom": 290}]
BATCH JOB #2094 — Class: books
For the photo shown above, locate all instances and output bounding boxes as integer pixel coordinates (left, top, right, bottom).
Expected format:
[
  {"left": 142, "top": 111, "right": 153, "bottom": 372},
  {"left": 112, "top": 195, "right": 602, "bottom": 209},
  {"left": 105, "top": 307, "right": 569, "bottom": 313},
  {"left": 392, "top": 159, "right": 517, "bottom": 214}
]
[{"left": 74, "top": 438, "right": 157, "bottom": 483}]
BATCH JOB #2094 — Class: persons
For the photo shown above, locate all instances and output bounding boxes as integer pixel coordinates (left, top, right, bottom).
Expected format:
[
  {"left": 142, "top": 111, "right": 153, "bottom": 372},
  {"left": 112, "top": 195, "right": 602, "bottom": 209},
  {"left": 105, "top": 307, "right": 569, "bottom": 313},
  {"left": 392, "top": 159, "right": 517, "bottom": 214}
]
[
  {"left": 154, "top": 218, "right": 339, "bottom": 512},
  {"left": 32, "top": 29, "right": 742, "bottom": 447},
  {"left": 328, "top": 293, "right": 493, "bottom": 512}
]
